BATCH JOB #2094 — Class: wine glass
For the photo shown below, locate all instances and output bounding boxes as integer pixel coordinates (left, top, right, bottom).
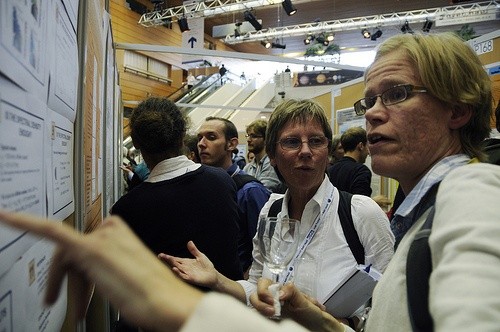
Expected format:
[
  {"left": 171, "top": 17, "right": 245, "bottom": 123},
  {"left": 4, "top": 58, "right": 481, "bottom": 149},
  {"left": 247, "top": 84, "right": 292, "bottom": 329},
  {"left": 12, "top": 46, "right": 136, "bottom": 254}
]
[{"left": 258, "top": 217, "right": 300, "bottom": 320}]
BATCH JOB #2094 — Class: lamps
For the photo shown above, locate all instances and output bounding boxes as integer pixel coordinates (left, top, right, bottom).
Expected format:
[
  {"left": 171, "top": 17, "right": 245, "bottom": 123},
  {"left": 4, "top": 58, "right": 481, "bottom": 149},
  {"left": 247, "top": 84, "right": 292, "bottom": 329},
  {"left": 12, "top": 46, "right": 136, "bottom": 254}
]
[{"left": 177, "top": 0, "right": 433, "bottom": 46}]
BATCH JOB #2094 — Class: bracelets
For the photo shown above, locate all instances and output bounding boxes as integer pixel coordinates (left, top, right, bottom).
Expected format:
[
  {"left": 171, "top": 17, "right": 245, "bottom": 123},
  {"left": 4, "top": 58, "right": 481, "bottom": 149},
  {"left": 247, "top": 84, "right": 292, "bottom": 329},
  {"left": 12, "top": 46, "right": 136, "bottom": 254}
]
[{"left": 348, "top": 317, "right": 357, "bottom": 331}]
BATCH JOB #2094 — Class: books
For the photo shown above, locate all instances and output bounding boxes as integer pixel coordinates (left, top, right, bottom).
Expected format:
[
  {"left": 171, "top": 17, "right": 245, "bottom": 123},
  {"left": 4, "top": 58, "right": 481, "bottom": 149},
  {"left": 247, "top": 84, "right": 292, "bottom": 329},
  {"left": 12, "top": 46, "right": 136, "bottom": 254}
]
[{"left": 320, "top": 264, "right": 383, "bottom": 319}]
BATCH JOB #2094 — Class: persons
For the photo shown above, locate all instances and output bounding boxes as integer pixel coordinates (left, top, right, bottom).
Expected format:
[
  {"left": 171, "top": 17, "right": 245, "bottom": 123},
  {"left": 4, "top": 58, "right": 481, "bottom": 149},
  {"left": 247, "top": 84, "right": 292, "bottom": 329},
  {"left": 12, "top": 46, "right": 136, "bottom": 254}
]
[
  {"left": 327, "top": 135, "right": 392, "bottom": 208},
  {"left": 186, "top": 70, "right": 195, "bottom": 96},
  {"left": 0, "top": 31, "right": 500, "bottom": 332},
  {"left": 109, "top": 95, "right": 238, "bottom": 295},
  {"left": 241, "top": 120, "right": 281, "bottom": 194},
  {"left": 326, "top": 126, "right": 373, "bottom": 197},
  {"left": 116, "top": 133, "right": 245, "bottom": 190},
  {"left": 285, "top": 65, "right": 290, "bottom": 72},
  {"left": 156, "top": 98, "right": 396, "bottom": 332},
  {"left": 218, "top": 62, "right": 226, "bottom": 85},
  {"left": 242, "top": 71, "right": 245, "bottom": 78},
  {"left": 247, "top": 152, "right": 256, "bottom": 165},
  {"left": 196, "top": 116, "right": 273, "bottom": 238}
]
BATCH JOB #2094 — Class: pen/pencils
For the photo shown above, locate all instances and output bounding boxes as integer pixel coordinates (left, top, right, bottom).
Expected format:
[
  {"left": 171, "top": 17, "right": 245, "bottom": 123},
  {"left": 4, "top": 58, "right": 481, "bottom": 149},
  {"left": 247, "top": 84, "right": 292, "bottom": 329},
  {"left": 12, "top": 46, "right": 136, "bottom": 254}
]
[
  {"left": 364, "top": 264, "right": 373, "bottom": 273},
  {"left": 124, "top": 165, "right": 132, "bottom": 168}
]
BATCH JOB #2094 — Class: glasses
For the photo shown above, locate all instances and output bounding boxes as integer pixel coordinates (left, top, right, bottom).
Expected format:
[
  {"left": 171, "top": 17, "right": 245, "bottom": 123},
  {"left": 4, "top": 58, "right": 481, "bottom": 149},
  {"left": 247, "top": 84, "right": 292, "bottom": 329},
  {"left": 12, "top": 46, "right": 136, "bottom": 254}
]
[
  {"left": 353, "top": 84, "right": 430, "bottom": 116},
  {"left": 245, "top": 134, "right": 260, "bottom": 139},
  {"left": 273, "top": 136, "right": 331, "bottom": 153}
]
